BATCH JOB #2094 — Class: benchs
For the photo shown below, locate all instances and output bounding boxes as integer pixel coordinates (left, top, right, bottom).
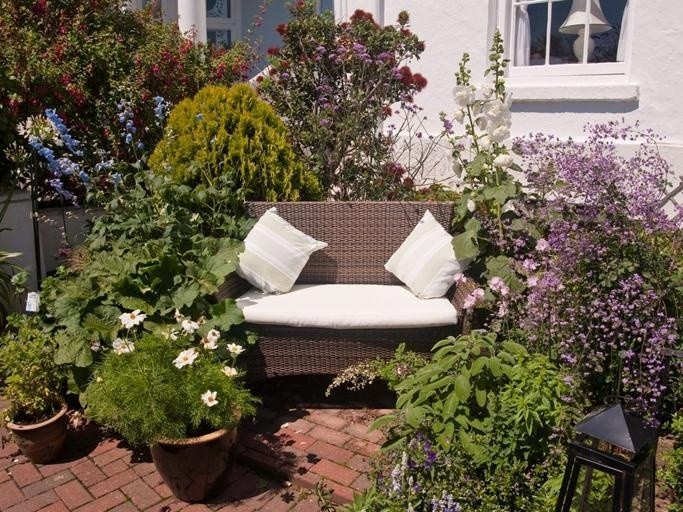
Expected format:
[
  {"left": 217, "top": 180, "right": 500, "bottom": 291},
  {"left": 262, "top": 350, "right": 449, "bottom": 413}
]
[{"left": 211, "top": 201, "right": 496, "bottom": 379}]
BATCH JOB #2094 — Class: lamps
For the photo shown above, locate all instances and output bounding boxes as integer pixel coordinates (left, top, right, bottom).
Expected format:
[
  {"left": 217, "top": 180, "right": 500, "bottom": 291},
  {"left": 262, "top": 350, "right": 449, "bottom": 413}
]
[{"left": 559, "top": 0, "right": 612, "bottom": 63}]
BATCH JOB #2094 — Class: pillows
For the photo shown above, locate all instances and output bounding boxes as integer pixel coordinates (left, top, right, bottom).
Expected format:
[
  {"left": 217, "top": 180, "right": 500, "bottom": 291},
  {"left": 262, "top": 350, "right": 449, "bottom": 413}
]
[
  {"left": 384, "top": 208, "right": 463, "bottom": 299},
  {"left": 229, "top": 207, "right": 329, "bottom": 294}
]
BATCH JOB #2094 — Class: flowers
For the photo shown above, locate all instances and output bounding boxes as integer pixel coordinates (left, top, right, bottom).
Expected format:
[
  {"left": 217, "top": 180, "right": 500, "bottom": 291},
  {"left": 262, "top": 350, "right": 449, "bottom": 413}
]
[{"left": 80, "top": 307, "right": 264, "bottom": 446}]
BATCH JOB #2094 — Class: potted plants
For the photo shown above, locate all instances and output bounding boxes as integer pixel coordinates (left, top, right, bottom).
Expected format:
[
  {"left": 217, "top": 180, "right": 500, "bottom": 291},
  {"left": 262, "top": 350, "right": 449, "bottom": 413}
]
[{"left": 1, "top": 312, "right": 68, "bottom": 463}]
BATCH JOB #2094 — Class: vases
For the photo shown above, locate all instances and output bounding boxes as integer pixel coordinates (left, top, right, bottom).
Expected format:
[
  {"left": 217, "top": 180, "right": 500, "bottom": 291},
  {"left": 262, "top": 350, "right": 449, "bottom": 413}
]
[{"left": 146, "top": 400, "right": 244, "bottom": 503}]
